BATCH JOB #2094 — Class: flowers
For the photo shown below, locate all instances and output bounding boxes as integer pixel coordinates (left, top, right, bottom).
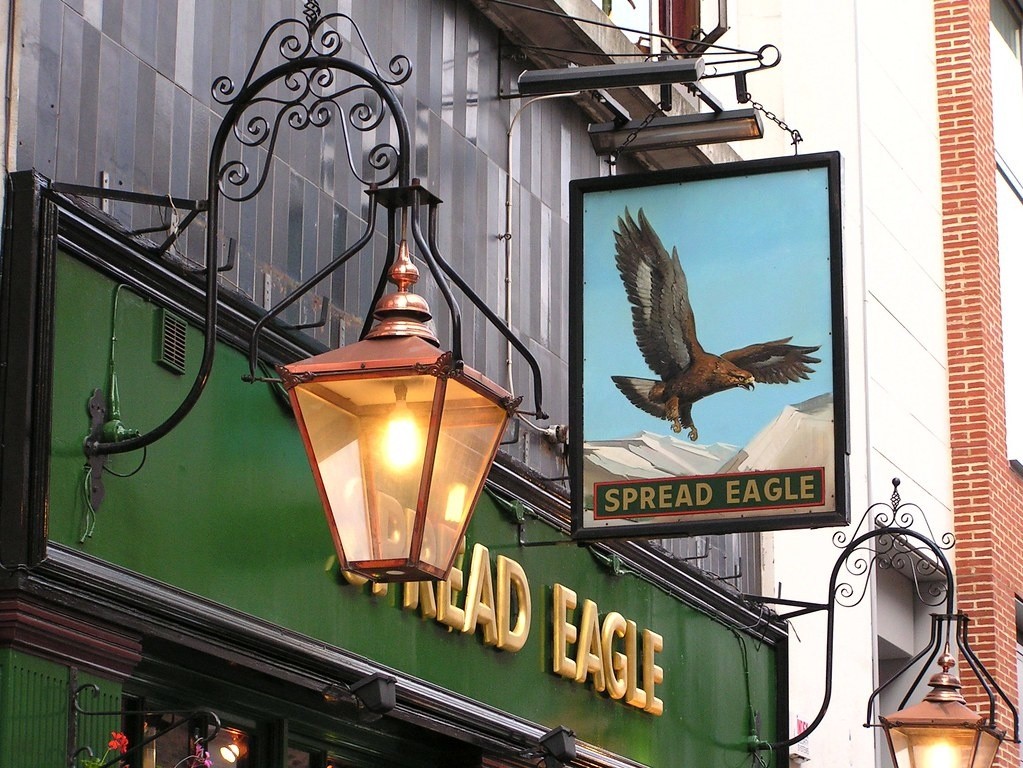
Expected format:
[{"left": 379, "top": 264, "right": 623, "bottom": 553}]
[
  {"left": 174, "top": 743, "right": 213, "bottom": 768},
  {"left": 78, "top": 731, "right": 130, "bottom": 768}
]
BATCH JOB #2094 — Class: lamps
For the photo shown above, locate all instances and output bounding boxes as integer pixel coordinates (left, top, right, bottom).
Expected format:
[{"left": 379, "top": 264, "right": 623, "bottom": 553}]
[
  {"left": 498, "top": 0, "right": 781, "bottom": 156},
  {"left": 519, "top": 725, "right": 577, "bottom": 761},
  {"left": 336, "top": 671, "right": 397, "bottom": 714},
  {"left": 220, "top": 745, "right": 247, "bottom": 768},
  {"left": 84, "top": 0, "right": 549, "bottom": 579},
  {"left": 752, "top": 478, "right": 1021, "bottom": 768}
]
